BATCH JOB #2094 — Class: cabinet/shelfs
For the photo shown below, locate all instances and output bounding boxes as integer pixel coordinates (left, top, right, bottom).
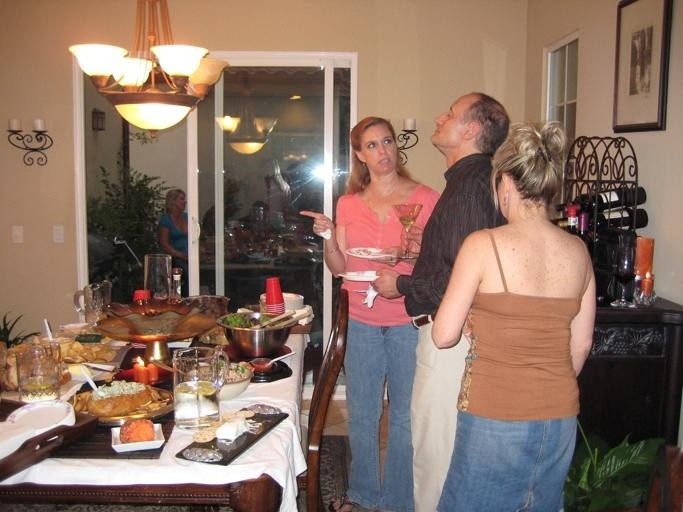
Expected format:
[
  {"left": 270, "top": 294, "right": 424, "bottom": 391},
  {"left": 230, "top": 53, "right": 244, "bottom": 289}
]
[{"left": 572, "top": 293, "right": 683, "bottom": 512}]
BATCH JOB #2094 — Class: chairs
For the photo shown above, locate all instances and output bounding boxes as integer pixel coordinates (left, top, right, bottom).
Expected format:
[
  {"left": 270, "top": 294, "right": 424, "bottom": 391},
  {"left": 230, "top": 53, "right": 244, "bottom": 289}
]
[{"left": 292, "top": 281, "right": 347, "bottom": 511}]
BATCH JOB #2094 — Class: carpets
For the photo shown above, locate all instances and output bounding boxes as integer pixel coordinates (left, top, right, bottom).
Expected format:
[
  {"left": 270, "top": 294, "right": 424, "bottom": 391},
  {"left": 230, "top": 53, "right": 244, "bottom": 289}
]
[{"left": 0, "top": 432, "right": 355, "bottom": 512}]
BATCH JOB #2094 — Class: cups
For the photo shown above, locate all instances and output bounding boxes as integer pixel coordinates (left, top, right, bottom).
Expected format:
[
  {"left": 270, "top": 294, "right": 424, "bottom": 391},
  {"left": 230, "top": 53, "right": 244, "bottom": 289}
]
[
  {"left": 250, "top": 357, "right": 273, "bottom": 373},
  {"left": 636, "top": 236, "right": 656, "bottom": 281},
  {"left": 72, "top": 281, "right": 112, "bottom": 324},
  {"left": 265, "top": 277, "right": 285, "bottom": 314},
  {"left": 13, "top": 340, "right": 69, "bottom": 402},
  {"left": 171, "top": 348, "right": 220, "bottom": 429},
  {"left": 144, "top": 253, "right": 170, "bottom": 302},
  {"left": 132, "top": 290, "right": 150, "bottom": 302},
  {"left": 636, "top": 273, "right": 656, "bottom": 307}
]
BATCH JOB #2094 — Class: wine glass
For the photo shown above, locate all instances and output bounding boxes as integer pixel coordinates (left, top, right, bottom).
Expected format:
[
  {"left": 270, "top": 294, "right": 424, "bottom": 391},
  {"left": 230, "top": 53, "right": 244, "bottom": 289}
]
[
  {"left": 389, "top": 204, "right": 423, "bottom": 259},
  {"left": 609, "top": 235, "right": 636, "bottom": 307}
]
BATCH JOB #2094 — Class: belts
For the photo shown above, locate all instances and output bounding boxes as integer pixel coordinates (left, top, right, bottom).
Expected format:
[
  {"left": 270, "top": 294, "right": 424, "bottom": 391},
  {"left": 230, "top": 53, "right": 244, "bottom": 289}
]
[{"left": 411, "top": 312, "right": 437, "bottom": 329}]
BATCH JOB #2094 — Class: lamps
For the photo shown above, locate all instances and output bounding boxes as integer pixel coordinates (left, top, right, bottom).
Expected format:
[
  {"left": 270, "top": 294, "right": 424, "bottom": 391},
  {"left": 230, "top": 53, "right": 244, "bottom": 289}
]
[
  {"left": 65, "top": 1, "right": 231, "bottom": 137},
  {"left": 215, "top": 68, "right": 277, "bottom": 158},
  {"left": 92, "top": 106, "right": 106, "bottom": 131}
]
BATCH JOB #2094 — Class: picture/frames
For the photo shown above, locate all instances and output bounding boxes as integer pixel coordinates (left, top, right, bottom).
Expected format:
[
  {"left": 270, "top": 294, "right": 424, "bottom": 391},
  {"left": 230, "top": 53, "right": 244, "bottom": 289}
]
[{"left": 610, "top": 0, "right": 677, "bottom": 131}]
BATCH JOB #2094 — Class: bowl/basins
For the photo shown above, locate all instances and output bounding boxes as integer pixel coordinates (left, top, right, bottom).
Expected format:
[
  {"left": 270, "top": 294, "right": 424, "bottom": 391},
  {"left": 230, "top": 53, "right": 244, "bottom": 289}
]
[
  {"left": 213, "top": 311, "right": 298, "bottom": 357},
  {"left": 216, "top": 361, "right": 252, "bottom": 402}
]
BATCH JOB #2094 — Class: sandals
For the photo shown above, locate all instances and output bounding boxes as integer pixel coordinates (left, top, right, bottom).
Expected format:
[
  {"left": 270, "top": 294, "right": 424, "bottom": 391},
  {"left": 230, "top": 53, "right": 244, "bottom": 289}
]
[{"left": 327, "top": 500, "right": 357, "bottom": 512}]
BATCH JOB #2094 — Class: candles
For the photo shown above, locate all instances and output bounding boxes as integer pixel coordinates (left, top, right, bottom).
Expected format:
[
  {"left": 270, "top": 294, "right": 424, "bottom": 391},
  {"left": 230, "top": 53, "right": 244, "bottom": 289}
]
[
  {"left": 133, "top": 363, "right": 142, "bottom": 381},
  {"left": 638, "top": 272, "right": 654, "bottom": 298},
  {"left": 402, "top": 116, "right": 416, "bottom": 129},
  {"left": 8, "top": 118, "right": 21, "bottom": 129},
  {"left": 31, "top": 118, "right": 45, "bottom": 130},
  {"left": 136, "top": 366, "right": 149, "bottom": 384},
  {"left": 148, "top": 364, "right": 159, "bottom": 382}
]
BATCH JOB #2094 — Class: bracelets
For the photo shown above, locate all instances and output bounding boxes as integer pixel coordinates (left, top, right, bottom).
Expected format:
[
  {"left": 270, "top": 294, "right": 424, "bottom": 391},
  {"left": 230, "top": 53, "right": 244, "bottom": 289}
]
[{"left": 326, "top": 244, "right": 339, "bottom": 254}]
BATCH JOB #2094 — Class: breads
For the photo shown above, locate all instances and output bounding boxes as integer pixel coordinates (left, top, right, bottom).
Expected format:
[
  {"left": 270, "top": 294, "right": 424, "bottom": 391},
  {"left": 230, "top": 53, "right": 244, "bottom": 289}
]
[
  {"left": 87, "top": 384, "right": 153, "bottom": 417},
  {"left": 3, "top": 343, "right": 71, "bottom": 391},
  {"left": 120, "top": 419, "right": 155, "bottom": 443}
]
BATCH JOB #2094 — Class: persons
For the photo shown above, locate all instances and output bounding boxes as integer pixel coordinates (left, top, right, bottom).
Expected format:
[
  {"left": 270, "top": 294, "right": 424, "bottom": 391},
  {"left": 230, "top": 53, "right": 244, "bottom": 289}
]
[
  {"left": 235, "top": 199, "right": 270, "bottom": 228},
  {"left": 432, "top": 118, "right": 598, "bottom": 512},
  {"left": 155, "top": 187, "right": 209, "bottom": 295},
  {"left": 198, "top": 204, "right": 230, "bottom": 246},
  {"left": 297, "top": 113, "right": 442, "bottom": 512},
  {"left": 369, "top": 90, "right": 515, "bottom": 512}
]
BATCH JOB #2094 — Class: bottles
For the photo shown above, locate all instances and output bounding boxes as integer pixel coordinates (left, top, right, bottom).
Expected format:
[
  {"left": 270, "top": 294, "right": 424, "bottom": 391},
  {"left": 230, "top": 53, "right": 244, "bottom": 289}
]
[
  {"left": 555, "top": 188, "right": 648, "bottom": 242},
  {"left": 172, "top": 273, "right": 182, "bottom": 298}
]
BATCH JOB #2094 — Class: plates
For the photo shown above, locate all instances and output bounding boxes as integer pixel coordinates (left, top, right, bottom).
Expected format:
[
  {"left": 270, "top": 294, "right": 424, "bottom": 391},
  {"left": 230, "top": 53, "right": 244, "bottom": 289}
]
[
  {"left": 336, "top": 271, "right": 379, "bottom": 282},
  {"left": 0, "top": 400, "right": 76, "bottom": 456},
  {"left": 109, "top": 424, "right": 165, "bottom": 452},
  {"left": 344, "top": 247, "right": 386, "bottom": 261}
]
